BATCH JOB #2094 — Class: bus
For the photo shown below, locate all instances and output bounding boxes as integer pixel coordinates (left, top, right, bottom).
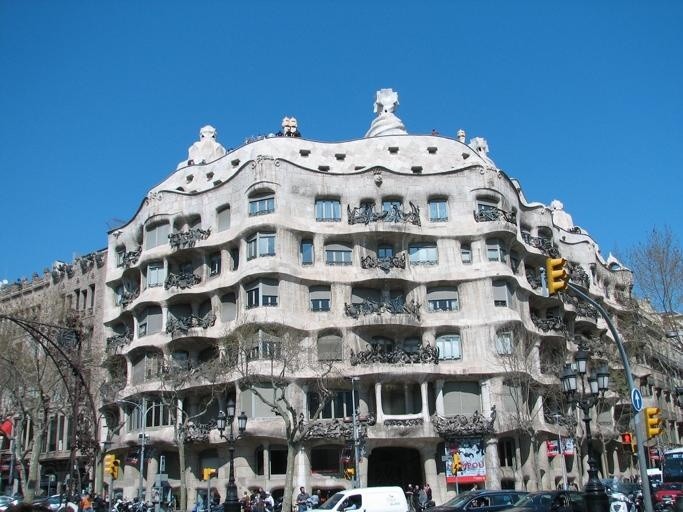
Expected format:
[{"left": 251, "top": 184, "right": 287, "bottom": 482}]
[{"left": 661, "top": 449, "right": 682, "bottom": 481}]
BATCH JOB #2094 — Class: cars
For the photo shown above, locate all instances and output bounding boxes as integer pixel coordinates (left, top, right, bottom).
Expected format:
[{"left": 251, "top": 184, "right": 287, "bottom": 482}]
[
  {"left": 0, "top": 492, "right": 79, "bottom": 512},
  {"left": 423, "top": 481, "right": 683, "bottom": 512}
]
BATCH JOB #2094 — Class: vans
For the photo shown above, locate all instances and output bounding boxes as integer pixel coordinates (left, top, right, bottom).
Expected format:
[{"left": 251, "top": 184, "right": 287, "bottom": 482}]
[{"left": 312, "top": 485, "right": 409, "bottom": 511}]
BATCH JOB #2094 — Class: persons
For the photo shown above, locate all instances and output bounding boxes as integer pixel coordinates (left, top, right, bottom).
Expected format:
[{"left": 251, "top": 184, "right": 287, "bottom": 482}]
[
  {"left": 479, "top": 498, "right": 487, "bottom": 507},
  {"left": 210, "top": 488, "right": 220, "bottom": 512},
  {"left": 471, "top": 483, "right": 478, "bottom": 491},
  {"left": 240, "top": 488, "right": 275, "bottom": 512},
  {"left": 570, "top": 483, "right": 578, "bottom": 491},
  {"left": 297, "top": 487, "right": 322, "bottom": 512},
  {"left": 172, "top": 495, "right": 176, "bottom": 512},
  {"left": 406, "top": 484, "right": 432, "bottom": 508},
  {"left": 340, "top": 498, "right": 356, "bottom": 512},
  {"left": 80, "top": 491, "right": 93, "bottom": 512}
]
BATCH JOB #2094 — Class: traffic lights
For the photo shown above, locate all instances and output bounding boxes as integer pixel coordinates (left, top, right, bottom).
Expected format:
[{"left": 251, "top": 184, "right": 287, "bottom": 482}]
[
  {"left": 546, "top": 258, "right": 566, "bottom": 296},
  {"left": 346, "top": 468, "right": 354, "bottom": 475},
  {"left": 644, "top": 407, "right": 663, "bottom": 439},
  {"left": 620, "top": 432, "right": 637, "bottom": 453},
  {"left": 103, "top": 454, "right": 119, "bottom": 480},
  {"left": 451, "top": 453, "right": 462, "bottom": 475},
  {"left": 203, "top": 468, "right": 216, "bottom": 481}
]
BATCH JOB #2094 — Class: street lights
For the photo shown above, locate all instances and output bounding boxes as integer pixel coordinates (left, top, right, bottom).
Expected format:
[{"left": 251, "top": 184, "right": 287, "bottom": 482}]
[
  {"left": 116, "top": 399, "right": 169, "bottom": 501},
  {"left": 351, "top": 376, "right": 360, "bottom": 488},
  {"left": 560, "top": 345, "right": 611, "bottom": 511},
  {"left": 216, "top": 399, "right": 247, "bottom": 511}
]
